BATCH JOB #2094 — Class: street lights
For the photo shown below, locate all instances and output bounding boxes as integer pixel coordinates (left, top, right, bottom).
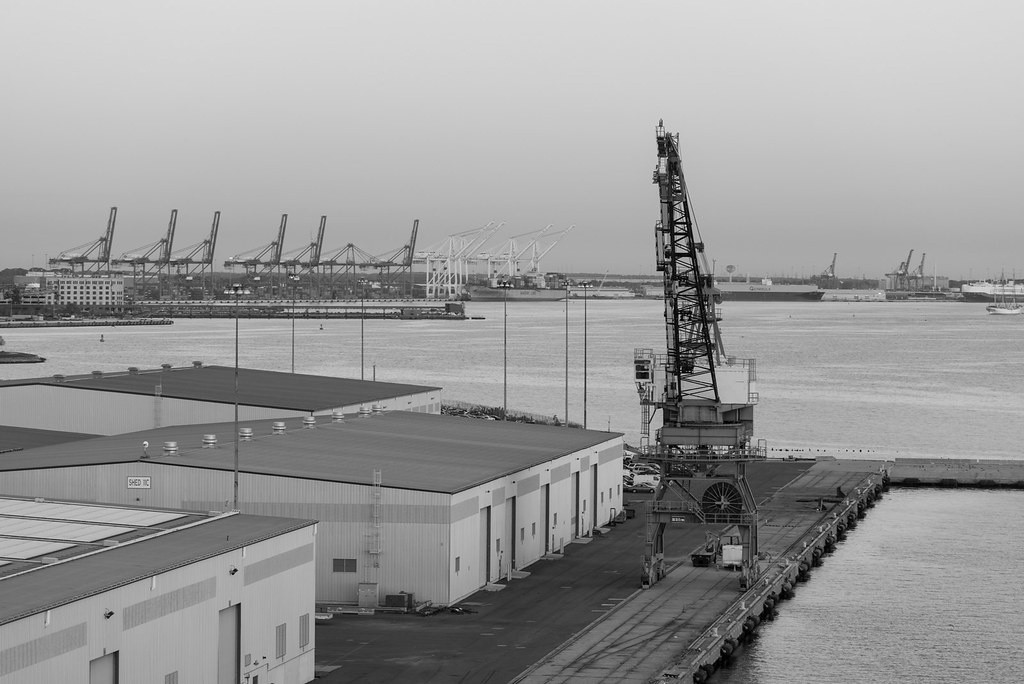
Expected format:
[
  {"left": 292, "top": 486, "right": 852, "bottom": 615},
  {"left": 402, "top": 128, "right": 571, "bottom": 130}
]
[
  {"left": 497, "top": 278, "right": 515, "bottom": 424},
  {"left": 578, "top": 280, "right": 594, "bottom": 427},
  {"left": 357, "top": 277, "right": 370, "bottom": 381},
  {"left": 561, "top": 277, "right": 573, "bottom": 426},
  {"left": 223, "top": 283, "right": 251, "bottom": 374},
  {"left": 288, "top": 273, "right": 299, "bottom": 371}
]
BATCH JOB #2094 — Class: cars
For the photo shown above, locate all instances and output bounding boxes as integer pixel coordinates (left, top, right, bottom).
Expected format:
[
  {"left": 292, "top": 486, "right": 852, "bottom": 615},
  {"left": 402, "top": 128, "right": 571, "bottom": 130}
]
[{"left": 623, "top": 463, "right": 674, "bottom": 493}]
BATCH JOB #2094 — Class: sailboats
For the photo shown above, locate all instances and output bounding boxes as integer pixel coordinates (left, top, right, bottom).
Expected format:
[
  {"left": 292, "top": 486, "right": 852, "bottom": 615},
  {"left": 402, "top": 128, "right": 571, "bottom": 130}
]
[{"left": 985, "top": 269, "right": 1023, "bottom": 315}]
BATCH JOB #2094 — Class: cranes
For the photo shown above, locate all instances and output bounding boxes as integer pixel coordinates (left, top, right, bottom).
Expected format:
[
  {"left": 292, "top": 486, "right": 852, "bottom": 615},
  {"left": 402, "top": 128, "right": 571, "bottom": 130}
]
[
  {"left": 634, "top": 116, "right": 763, "bottom": 592},
  {"left": 49, "top": 205, "right": 577, "bottom": 301},
  {"left": 817, "top": 252, "right": 839, "bottom": 289},
  {"left": 885, "top": 249, "right": 927, "bottom": 291}
]
